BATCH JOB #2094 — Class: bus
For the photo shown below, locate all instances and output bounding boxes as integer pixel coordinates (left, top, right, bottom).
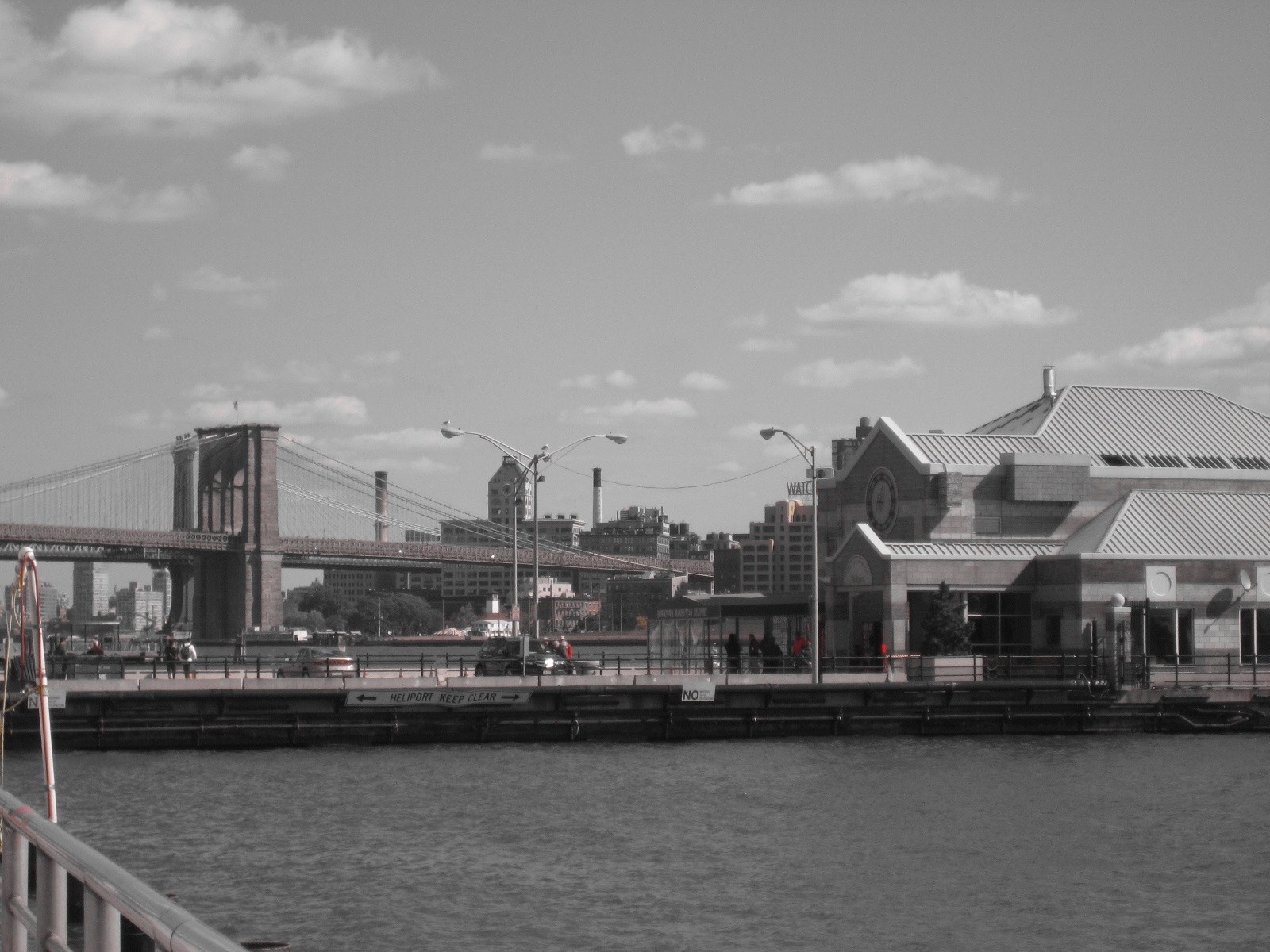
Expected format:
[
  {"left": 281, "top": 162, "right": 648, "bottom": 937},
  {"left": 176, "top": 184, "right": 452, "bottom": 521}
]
[{"left": 312, "top": 629, "right": 362, "bottom": 645}]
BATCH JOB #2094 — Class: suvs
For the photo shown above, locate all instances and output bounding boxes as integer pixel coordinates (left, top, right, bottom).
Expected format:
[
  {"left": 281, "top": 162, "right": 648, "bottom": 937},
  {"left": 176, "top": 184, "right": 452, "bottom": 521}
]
[{"left": 475, "top": 636, "right": 573, "bottom": 675}]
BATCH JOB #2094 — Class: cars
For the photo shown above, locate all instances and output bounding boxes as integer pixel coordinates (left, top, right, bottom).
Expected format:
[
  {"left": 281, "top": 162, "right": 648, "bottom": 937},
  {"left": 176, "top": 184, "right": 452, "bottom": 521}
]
[
  {"left": 277, "top": 647, "right": 356, "bottom": 678},
  {"left": 63, "top": 637, "right": 97, "bottom": 658}
]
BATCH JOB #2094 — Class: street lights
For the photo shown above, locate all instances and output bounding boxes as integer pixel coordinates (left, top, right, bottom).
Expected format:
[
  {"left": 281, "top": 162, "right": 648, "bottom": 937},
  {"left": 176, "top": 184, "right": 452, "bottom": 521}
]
[
  {"left": 439, "top": 418, "right": 628, "bottom": 640},
  {"left": 511, "top": 452, "right": 547, "bottom": 637},
  {"left": 759, "top": 427, "right": 819, "bottom": 684},
  {"left": 116, "top": 616, "right": 123, "bottom": 651}
]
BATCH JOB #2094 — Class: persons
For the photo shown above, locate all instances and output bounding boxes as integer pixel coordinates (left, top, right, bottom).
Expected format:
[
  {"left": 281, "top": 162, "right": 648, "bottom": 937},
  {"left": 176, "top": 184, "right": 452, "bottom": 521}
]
[
  {"left": 725, "top": 633, "right": 812, "bottom": 674},
  {"left": 162, "top": 639, "right": 179, "bottom": 680},
  {"left": 88, "top": 640, "right": 104, "bottom": 655},
  {"left": 179, "top": 637, "right": 197, "bottom": 679},
  {"left": 54, "top": 636, "right": 69, "bottom": 679},
  {"left": 538, "top": 636, "right": 573, "bottom": 662}
]
